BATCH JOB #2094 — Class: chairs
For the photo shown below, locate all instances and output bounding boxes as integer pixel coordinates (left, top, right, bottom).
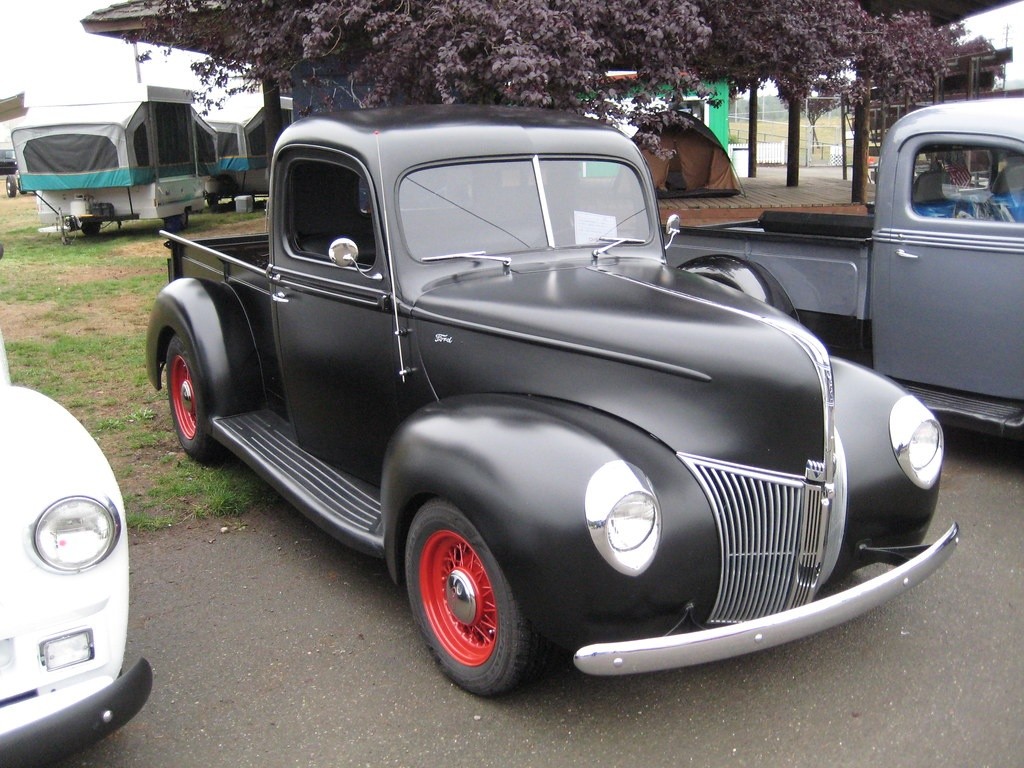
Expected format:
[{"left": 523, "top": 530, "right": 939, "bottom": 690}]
[
  {"left": 918, "top": 171, "right": 958, "bottom": 219},
  {"left": 987, "top": 162, "right": 1024, "bottom": 224}
]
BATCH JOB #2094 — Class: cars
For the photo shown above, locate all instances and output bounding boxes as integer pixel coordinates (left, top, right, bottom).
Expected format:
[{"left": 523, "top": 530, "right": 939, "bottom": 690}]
[
  {"left": 0, "top": 341, "right": 153, "bottom": 768},
  {"left": 143, "top": 97, "right": 961, "bottom": 700}
]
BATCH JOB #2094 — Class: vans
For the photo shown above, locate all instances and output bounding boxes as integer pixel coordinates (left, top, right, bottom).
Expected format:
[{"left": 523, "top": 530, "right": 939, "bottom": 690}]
[{"left": 0, "top": 149, "right": 18, "bottom": 175}]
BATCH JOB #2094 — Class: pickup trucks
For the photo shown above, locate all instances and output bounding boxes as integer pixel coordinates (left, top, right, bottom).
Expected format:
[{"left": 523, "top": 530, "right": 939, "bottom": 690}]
[{"left": 659, "top": 96, "right": 1024, "bottom": 441}]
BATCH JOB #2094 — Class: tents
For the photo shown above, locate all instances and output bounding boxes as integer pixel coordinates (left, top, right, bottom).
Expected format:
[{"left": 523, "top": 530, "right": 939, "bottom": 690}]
[{"left": 633, "top": 109, "right": 746, "bottom": 199}]
